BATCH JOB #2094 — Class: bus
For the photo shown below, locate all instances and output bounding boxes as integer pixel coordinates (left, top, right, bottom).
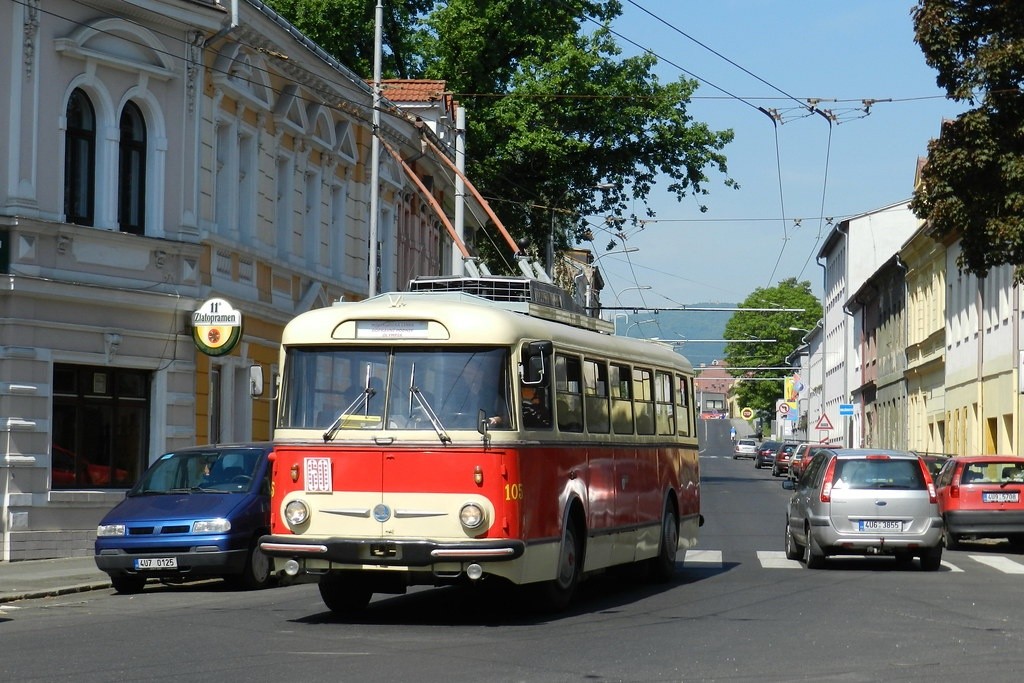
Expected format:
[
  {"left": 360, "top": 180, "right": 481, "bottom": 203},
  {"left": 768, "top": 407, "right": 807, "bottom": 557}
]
[{"left": 249, "top": 93, "right": 709, "bottom": 612}]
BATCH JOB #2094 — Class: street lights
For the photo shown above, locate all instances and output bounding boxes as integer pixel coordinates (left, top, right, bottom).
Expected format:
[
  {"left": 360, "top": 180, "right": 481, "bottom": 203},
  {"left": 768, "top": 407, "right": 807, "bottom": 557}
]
[
  {"left": 612, "top": 285, "right": 651, "bottom": 338},
  {"left": 585, "top": 246, "right": 640, "bottom": 322}
]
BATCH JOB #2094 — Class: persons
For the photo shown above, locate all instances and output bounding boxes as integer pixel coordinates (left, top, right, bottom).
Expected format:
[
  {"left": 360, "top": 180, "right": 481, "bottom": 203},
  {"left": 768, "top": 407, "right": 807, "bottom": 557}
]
[
  {"left": 334, "top": 377, "right": 434, "bottom": 430},
  {"left": 521, "top": 385, "right": 552, "bottom": 428},
  {"left": 730, "top": 427, "right": 737, "bottom": 441},
  {"left": 756, "top": 426, "right": 764, "bottom": 442},
  {"left": 444, "top": 356, "right": 509, "bottom": 428}
]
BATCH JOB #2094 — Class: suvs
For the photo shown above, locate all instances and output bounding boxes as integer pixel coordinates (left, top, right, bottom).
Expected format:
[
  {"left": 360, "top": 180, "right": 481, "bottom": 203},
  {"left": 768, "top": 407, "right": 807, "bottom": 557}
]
[{"left": 787, "top": 442, "right": 844, "bottom": 485}]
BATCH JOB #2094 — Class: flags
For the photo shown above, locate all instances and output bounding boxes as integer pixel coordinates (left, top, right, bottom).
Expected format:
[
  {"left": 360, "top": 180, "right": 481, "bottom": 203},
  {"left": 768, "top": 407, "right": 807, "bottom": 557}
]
[
  {"left": 784, "top": 378, "right": 797, "bottom": 409},
  {"left": 791, "top": 374, "right": 808, "bottom": 400}
]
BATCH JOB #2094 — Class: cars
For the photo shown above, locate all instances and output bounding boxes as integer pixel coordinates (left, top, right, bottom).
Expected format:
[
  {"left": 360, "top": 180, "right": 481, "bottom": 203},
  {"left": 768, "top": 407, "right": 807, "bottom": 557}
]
[
  {"left": 934, "top": 455, "right": 1024, "bottom": 549},
  {"left": 771, "top": 443, "right": 797, "bottom": 477},
  {"left": 755, "top": 441, "right": 786, "bottom": 471},
  {"left": 93, "top": 443, "right": 277, "bottom": 595},
  {"left": 914, "top": 450, "right": 954, "bottom": 488},
  {"left": 734, "top": 439, "right": 758, "bottom": 460},
  {"left": 783, "top": 448, "right": 947, "bottom": 572}
]
